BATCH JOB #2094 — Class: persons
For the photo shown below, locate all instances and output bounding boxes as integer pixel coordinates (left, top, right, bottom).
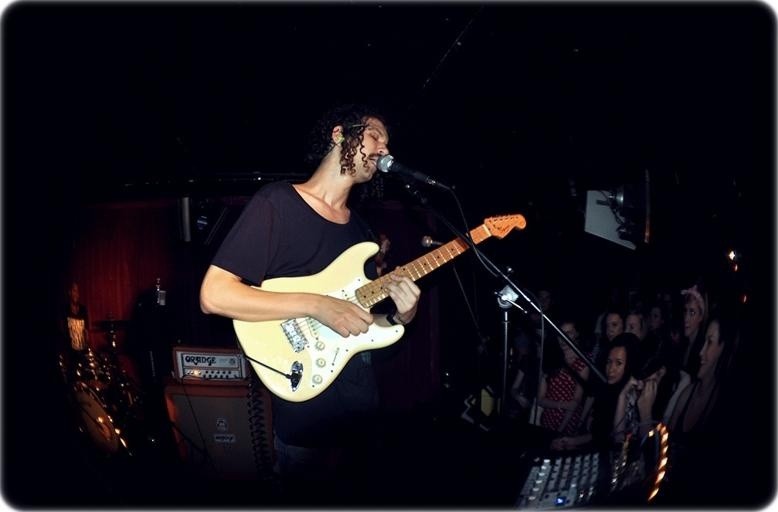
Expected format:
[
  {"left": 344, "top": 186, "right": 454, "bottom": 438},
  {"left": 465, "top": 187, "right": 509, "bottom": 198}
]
[
  {"left": 501, "top": 280, "right": 747, "bottom": 456},
  {"left": 198, "top": 101, "right": 426, "bottom": 505}
]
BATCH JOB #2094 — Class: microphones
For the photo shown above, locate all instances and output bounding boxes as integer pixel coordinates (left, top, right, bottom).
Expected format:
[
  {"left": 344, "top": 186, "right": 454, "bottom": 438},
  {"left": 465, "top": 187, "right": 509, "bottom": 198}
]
[
  {"left": 422, "top": 236, "right": 442, "bottom": 249},
  {"left": 376, "top": 153, "right": 439, "bottom": 189}
]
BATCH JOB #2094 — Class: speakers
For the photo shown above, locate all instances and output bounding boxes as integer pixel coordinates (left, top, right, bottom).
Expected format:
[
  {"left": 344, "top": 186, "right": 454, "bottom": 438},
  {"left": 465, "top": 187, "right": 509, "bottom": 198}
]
[{"left": 164, "top": 384, "right": 272, "bottom": 476}]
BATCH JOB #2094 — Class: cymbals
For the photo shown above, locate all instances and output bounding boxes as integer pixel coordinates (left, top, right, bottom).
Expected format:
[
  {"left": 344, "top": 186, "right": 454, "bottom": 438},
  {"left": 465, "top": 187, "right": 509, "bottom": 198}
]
[{"left": 93, "top": 320, "right": 128, "bottom": 330}]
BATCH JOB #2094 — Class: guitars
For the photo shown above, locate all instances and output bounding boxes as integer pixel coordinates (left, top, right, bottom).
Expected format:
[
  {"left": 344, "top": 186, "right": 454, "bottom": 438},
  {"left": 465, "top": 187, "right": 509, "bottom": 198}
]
[{"left": 233, "top": 213, "right": 526, "bottom": 401}]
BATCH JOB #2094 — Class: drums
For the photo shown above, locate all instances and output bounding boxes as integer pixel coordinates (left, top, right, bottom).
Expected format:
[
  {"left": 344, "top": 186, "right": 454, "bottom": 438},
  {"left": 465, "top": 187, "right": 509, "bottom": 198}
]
[{"left": 71, "top": 373, "right": 139, "bottom": 454}]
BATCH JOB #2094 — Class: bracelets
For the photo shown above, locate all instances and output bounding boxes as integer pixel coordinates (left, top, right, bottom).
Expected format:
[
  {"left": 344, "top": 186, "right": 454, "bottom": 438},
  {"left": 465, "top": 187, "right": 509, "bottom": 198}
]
[{"left": 385, "top": 311, "right": 401, "bottom": 327}]
